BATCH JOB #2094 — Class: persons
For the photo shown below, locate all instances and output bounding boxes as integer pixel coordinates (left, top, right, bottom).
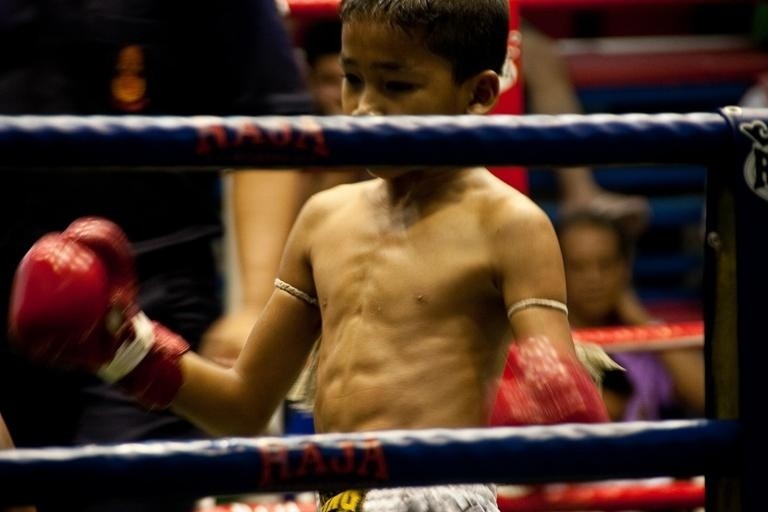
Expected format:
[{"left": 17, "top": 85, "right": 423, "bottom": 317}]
[{"left": 0, "top": 2, "right": 705, "bottom": 512}]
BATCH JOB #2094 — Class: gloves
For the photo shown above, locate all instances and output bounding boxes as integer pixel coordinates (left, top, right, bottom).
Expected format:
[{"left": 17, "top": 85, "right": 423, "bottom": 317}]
[
  {"left": 492, "top": 341, "right": 610, "bottom": 432},
  {"left": 11, "top": 214, "right": 192, "bottom": 414}
]
